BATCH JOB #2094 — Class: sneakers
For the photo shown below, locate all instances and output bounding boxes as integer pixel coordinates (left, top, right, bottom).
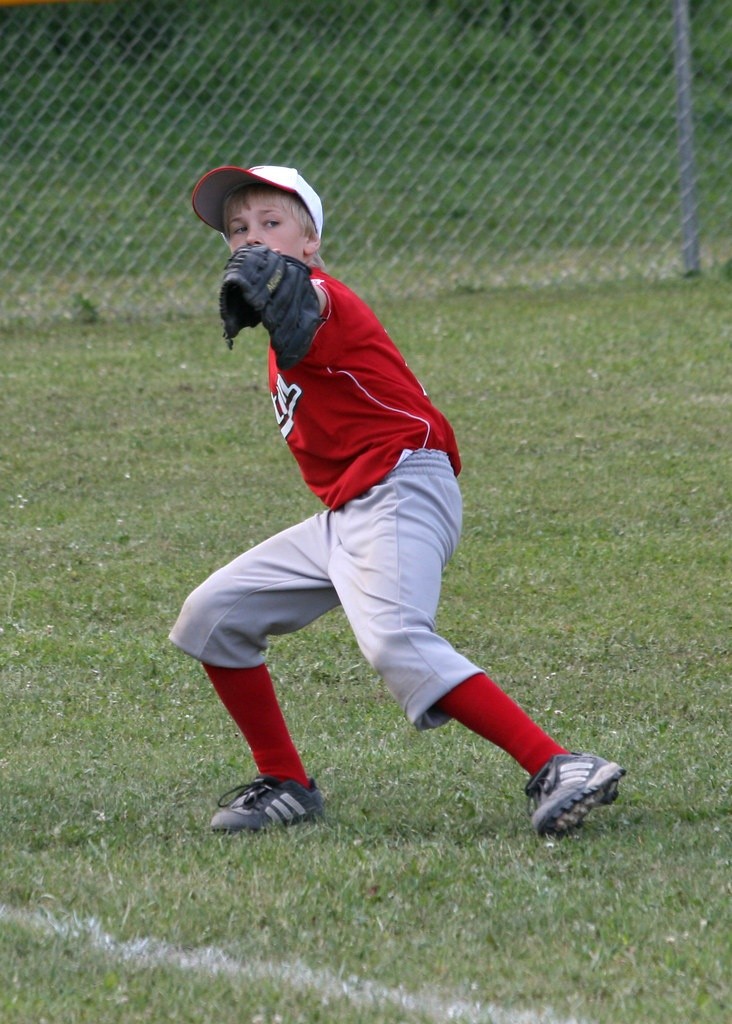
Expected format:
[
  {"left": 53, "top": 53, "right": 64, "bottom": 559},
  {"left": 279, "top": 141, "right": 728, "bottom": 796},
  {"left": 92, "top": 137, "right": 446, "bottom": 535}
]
[
  {"left": 210, "top": 773, "right": 322, "bottom": 833},
  {"left": 525, "top": 750, "right": 627, "bottom": 836}
]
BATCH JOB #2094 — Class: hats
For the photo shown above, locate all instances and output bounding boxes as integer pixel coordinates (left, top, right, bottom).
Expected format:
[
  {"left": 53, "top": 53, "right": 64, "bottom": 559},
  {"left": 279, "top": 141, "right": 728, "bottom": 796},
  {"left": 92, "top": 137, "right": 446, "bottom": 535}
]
[{"left": 193, "top": 166, "right": 323, "bottom": 247}]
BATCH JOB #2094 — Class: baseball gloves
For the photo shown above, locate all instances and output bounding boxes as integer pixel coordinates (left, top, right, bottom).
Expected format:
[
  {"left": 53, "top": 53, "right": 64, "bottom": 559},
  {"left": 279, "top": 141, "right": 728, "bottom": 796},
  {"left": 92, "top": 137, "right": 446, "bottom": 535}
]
[{"left": 217, "top": 241, "right": 327, "bottom": 374}]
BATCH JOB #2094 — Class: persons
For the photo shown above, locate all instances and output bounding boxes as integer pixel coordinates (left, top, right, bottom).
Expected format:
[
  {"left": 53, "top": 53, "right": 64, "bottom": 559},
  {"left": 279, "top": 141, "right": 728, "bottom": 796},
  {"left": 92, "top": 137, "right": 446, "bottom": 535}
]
[{"left": 168, "top": 165, "right": 627, "bottom": 838}]
